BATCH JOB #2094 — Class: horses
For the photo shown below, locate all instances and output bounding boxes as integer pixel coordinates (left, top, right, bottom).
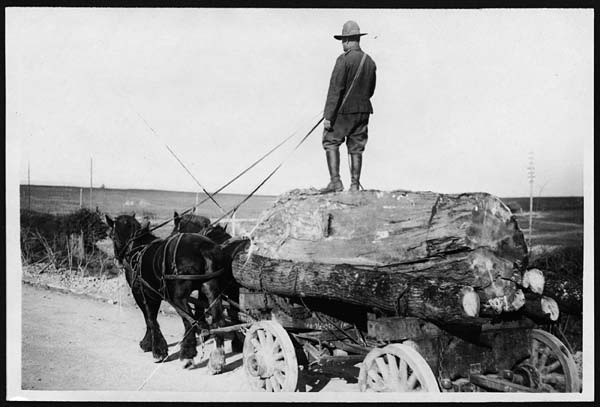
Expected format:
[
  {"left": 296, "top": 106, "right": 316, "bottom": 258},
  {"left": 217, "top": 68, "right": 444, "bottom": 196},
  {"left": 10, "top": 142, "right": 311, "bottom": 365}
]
[
  {"left": 169, "top": 206, "right": 253, "bottom": 355},
  {"left": 103, "top": 208, "right": 234, "bottom": 376}
]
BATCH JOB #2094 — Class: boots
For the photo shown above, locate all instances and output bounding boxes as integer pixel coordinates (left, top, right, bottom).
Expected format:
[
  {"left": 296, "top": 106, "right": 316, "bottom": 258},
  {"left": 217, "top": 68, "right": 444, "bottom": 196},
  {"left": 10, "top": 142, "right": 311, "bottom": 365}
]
[
  {"left": 319, "top": 150, "right": 344, "bottom": 192},
  {"left": 348, "top": 153, "right": 363, "bottom": 192}
]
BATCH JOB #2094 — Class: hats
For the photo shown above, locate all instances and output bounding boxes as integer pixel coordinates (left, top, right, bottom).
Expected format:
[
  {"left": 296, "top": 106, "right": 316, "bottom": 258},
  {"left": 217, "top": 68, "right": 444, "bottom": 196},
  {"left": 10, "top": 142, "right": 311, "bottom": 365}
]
[{"left": 334, "top": 22, "right": 368, "bottom": 40}]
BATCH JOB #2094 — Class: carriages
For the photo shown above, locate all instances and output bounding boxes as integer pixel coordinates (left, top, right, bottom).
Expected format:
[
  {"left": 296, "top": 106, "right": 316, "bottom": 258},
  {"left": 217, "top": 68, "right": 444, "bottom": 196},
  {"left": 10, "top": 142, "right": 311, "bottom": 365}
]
[{"left": 105, "top": 210, "right": 581, "bottom": 393}]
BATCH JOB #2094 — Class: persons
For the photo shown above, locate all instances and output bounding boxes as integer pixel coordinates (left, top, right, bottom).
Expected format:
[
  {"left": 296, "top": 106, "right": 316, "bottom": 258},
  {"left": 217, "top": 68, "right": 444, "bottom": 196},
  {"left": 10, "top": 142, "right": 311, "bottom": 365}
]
[{"left": 319, "top": 21, "right": 376, "bottom": 194}]
